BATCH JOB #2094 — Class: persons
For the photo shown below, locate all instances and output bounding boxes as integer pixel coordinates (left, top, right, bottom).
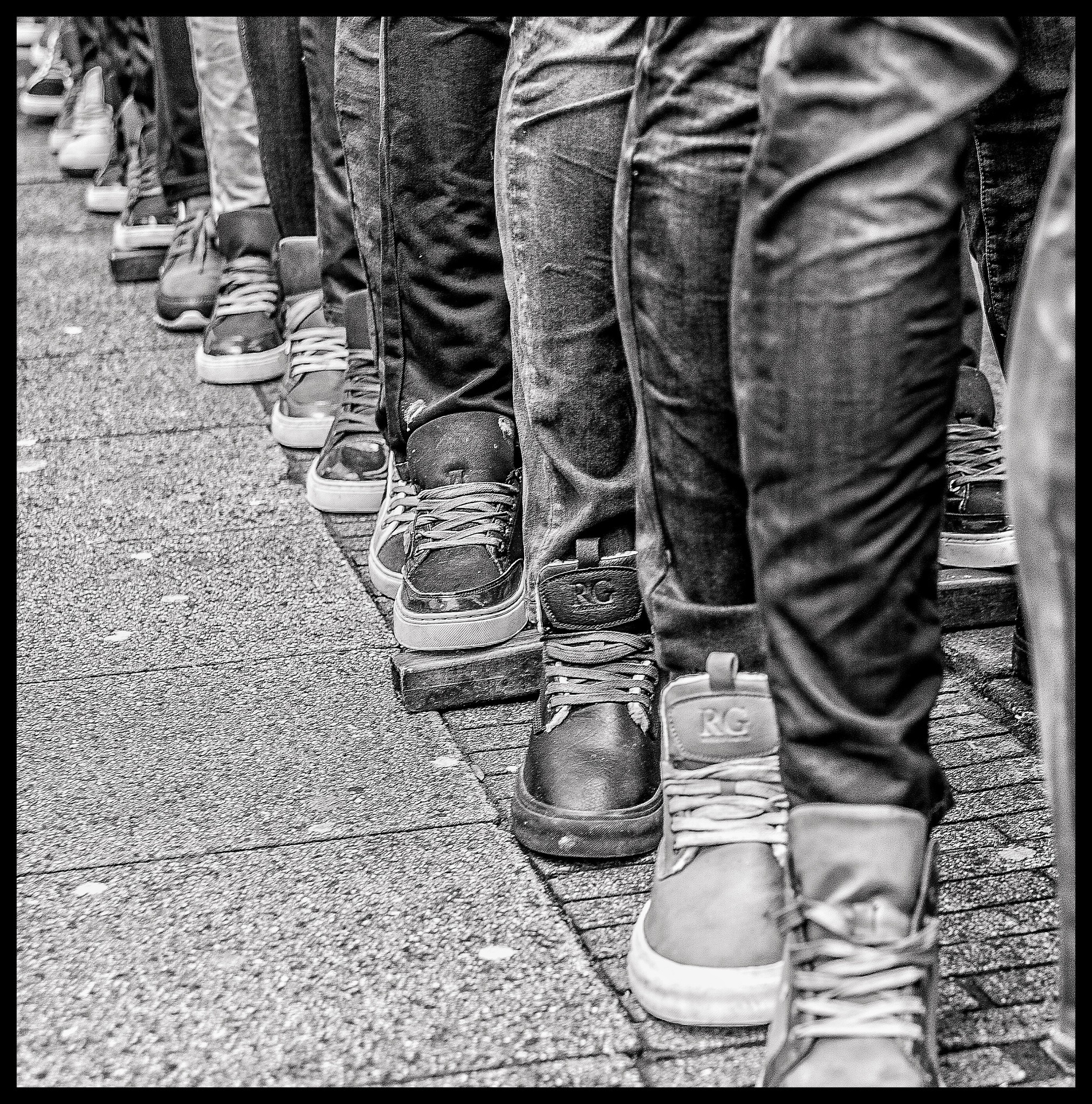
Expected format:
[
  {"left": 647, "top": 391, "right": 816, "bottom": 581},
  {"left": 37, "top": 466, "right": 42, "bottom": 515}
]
[
  {"left": 728, "top": 16, "right": 1076, "bottom": 1091},
  {"left": 235, "top": 22, "right": 352, "bottom": 449},
  {"left": 333, "top": 9, "right": 1018, "bottom": 657},
  {"left": 614, "top": 11, "right": 812, "bottom": 1034},
  {"left": 13, "top": 13, "right": 221, "bottom": 333},
  {"left": 188, "top": 10, "right": 286, "bottom": 387},
  {"left": 1001, "top": 95, "right": 1083, "bottom": 1091},
  {"left": 489, "top": 11, "right": 666, "bottom": 873}
]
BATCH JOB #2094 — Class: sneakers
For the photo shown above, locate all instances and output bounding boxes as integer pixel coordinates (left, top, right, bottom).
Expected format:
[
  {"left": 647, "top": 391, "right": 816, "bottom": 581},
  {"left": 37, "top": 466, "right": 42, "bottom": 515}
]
[
  {"left": 196, "top": 209, "right": 288, "bottom": 384},
  {"left": 48, "top": 81, "right": 80, "bottom": 152},
  {"left": 937, "top": 364, "right": 1021, "bottom": 569},
  {"left": 272, "top": 236, "right": 351, "bottom": 450},
  {"left": 759, "top": 803, "right": 938, "bottom": 1088},
  {"left": 627, "top": 650, "right": 794, "bottom": 1028},
  {"left": 511, "top": 539, "right": 662, "bottom": 862},
  {"left": 117, "top": 120, "right": 183, "bottom": 249},
  {"left": 154, "top": 196, "right": 224, "bottom": 330},
  {"left": 85, "top": 141, "right": 127, "bottom": 213},
  {"left": 367, "top": 451, "right": 422, "bottom": 601},
  {"left": 20, "top": 59, "right": 73, "bottom": 117},
  {"left": 394, "top": 411, "right": 529, "bottom": 649},
  {"left": 59, "top": 66, "right": 117, "bottom": 169},
  {"left": 306, "top": 290, "right": 392, "bottom": 513}
]
